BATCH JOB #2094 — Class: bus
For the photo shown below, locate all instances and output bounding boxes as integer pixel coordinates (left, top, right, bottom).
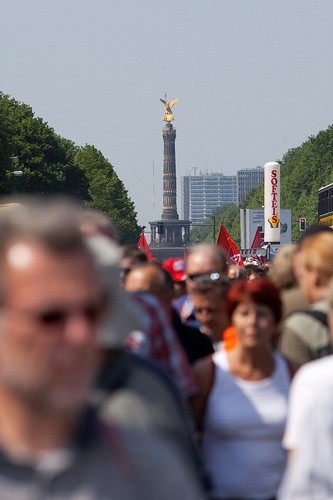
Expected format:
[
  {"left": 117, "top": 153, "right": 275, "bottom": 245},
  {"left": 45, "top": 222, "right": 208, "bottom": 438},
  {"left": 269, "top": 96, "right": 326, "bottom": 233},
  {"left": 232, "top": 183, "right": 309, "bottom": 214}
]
[{"left": 317, "top": 182, "right": 333, "bottom": 229}]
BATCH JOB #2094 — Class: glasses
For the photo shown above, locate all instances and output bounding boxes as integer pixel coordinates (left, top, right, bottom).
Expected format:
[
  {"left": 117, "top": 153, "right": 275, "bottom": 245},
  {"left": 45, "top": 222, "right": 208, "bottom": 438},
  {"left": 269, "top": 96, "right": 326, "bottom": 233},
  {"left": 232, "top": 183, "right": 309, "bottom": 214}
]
[
  {"left": 0, "top": 304, "right": 100, "bottom": 325},
  {"left": 118, "top": 268, "right": 130, "bottom": 274}
]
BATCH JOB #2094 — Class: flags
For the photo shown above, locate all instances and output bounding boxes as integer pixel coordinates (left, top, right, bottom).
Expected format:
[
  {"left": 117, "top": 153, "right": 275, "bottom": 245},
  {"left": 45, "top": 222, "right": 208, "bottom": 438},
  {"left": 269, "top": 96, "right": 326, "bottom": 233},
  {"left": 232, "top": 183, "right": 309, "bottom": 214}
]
[
  {"left": 136, "top": 232, "right": 155, "bottom": 262},
  {"left": 251, "top": 227, "right": 265, "bottom": 266},
  {"left": 216, "top": 222, "right": 244, "bottom": 268},
  {"left": 183, "top": 246, "right": 188, "bottom": 261}
]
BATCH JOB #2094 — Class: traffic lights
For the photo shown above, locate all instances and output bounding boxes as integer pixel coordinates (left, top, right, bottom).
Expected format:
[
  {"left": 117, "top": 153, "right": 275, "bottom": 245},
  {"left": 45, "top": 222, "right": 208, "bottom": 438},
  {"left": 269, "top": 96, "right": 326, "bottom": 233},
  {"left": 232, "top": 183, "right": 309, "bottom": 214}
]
[{"left": 299, "top": 218, "right": 306, "bottom": 231}]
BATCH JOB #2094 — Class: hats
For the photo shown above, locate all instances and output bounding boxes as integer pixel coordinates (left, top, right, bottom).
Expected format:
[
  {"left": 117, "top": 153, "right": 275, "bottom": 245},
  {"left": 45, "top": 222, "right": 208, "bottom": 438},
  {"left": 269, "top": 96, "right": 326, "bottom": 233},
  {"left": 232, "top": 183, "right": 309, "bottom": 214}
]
[{"left": 162, "top": 258, "right": 187, "bottom": 282}]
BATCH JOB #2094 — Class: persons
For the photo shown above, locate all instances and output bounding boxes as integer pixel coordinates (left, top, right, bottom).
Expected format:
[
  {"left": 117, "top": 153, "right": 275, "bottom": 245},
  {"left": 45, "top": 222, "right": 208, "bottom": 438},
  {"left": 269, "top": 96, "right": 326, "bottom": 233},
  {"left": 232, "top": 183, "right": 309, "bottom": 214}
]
[
  {"left": 181, "top": 275, "right": 290, "bottom": 500},
  {"left": 0, "top": 197, "right": 333, "bottom": 500},
  {"left": 0, "top": 198, "right": 205, "bottom": 500}
]
